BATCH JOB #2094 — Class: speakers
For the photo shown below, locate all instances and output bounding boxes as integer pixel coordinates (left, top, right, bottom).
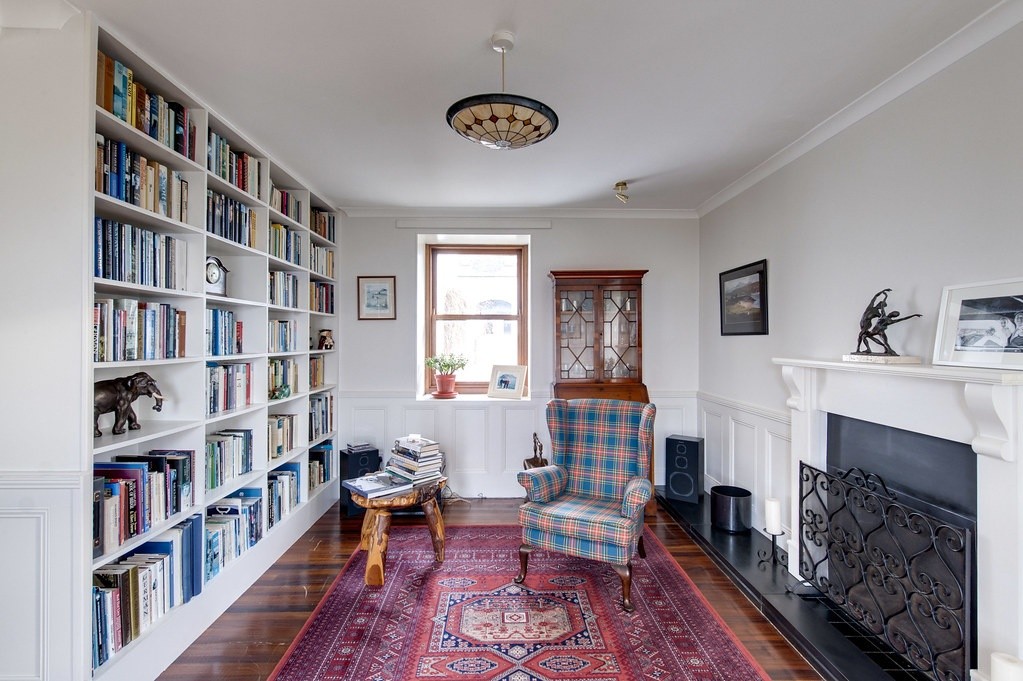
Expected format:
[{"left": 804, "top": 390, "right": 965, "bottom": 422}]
[
  {"left": 664, "top": 434, "right": 706, "bottom": 504},
  {"left": 340, "top": 447, "right": 378, "bottom": 516}
]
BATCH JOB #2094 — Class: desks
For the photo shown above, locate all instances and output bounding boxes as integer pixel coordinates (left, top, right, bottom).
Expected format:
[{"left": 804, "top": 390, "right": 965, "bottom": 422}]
[{"left": 352, "top": 477, "right": 448, "bottom": 587}]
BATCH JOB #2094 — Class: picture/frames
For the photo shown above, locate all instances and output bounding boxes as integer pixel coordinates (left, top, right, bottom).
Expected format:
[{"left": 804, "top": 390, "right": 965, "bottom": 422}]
[
  {"left": 487, "top": 364, "right": 528, "bottom": 399},
  {"left": 933, "top": 277, "right": 1023, "bottom": 371},
  {"left": 719, "top": 259, "right": 770, "bottom": 335},
  {"left": 357, "top": 276, "right": 396, "bottom": 320}
]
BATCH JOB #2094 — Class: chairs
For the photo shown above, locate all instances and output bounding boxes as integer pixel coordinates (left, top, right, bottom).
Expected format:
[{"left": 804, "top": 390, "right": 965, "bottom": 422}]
[{"left": 515, "top": 398, "right": 656, "bottom": 611}]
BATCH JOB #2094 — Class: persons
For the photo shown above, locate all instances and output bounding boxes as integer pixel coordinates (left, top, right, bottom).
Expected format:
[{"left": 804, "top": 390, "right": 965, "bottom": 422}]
[
  {"left": 856, "top": 289, "right": 924, "bottom": 354},
  {"left": 1000, "top": 310, "right": 1023, "bottom": 353}
]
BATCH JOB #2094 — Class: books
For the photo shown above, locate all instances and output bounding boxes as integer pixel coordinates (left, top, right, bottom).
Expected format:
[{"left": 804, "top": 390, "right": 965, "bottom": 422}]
[
  {"left": 92, "top": 51, "right": 335, "bottom": 671},
  {"left": 342, "top": 434, "right": 446, "bottom": 499}
]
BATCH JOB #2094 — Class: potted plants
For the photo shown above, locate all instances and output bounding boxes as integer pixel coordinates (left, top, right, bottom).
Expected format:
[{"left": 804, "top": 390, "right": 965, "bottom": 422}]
[{"left": 425, "top": 353, "right": 468, "bottom": 393}]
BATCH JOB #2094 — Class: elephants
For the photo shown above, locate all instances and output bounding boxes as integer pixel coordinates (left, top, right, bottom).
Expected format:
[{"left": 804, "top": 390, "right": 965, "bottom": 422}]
[{"left": 94, "top": 372, "right": 169, "bottom": 437}]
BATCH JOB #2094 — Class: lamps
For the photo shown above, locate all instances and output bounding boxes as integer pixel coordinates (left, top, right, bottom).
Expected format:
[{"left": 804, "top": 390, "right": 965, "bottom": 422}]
[
  {"left": 613, "top": 182, "right": 630, "bottom": 203},
  {"left": 445, "top": 31, "right": 559, "bottom": 149}
]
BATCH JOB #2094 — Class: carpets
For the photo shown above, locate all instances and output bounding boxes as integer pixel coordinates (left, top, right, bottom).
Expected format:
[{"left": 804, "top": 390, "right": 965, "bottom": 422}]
[{"left": 267, "top": 523, "right": 770, "bottom": 681}]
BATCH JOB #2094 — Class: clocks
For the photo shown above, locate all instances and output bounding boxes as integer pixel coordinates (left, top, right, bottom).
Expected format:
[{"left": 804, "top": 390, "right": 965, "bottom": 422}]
[{"left": 207, "top": 256, "right": 230, "bottom": 296}]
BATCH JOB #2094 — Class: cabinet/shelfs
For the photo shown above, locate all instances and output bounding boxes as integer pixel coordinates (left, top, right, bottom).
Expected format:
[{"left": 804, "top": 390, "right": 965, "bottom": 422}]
[
  {"left": 87, "top": 12, "right": 342, "bottom": 681},
  {"left": 547, "top": 269, "right": 656, "bottom": 516}
]
